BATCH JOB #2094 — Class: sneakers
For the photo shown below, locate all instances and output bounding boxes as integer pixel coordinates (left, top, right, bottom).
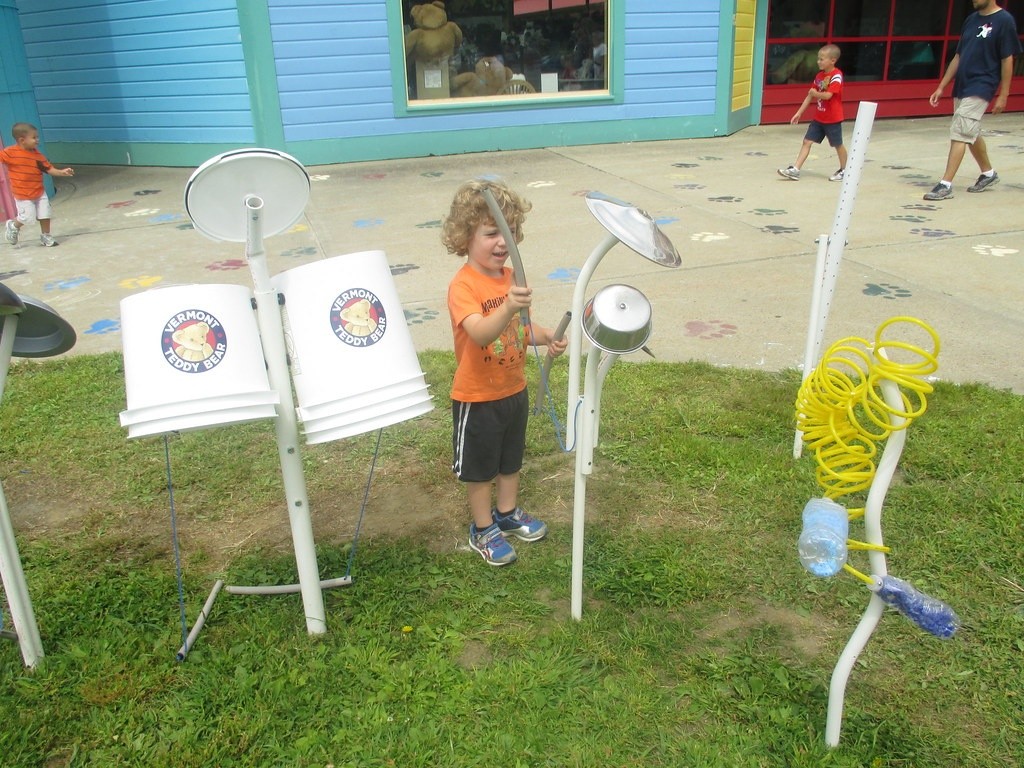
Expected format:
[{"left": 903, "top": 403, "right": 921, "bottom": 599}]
[
  {"left": 778, "top": 166, "right": 800, "bottom": 180},
  {"left": 829, "top": 167, "right": 845, "bottom": 182},
  {"left": 967, "top": 171, "right": 1000, "bottom": 192},
  {"left": 5, "top": 220, "right": 20, "bottom": 244},
  {"left": 492, "top": 506, "right": 547, "bottom": 541},
  {"left": 923, "top": 183, "right": 954, "bottom": 200},
  {"left": 469, "top": 522, "right": 516, "bottom": 566},
  {"left": 40, "top": 233, "right": 59, "bottom": 247}
]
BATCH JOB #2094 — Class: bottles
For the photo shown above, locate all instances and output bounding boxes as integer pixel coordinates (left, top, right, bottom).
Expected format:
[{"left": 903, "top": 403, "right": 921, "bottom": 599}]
[{"left": 797, "top": 498, "right": 849, "bottom": 578}]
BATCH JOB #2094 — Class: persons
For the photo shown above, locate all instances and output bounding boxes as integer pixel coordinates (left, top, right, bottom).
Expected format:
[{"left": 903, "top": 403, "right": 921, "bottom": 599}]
[
  {"left": 776, "top": 44, "right": 848, "bottom": 182},
  {"left": 441, "top": 179, "right": 569, "bottom": 566},
  {"left": 922, "top": 0, "right": 1017, "bottom": 201},
  {"left": 1, "top": 122, "right": 75, "bottom": 247}
]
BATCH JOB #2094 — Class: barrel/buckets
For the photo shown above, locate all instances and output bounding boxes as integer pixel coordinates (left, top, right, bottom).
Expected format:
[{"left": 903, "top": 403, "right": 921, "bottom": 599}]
[
  {"left": 269, "top": 250, "right": 435, "bottom": 445},
  {"left": 118, "top": 283, "right": 281, "bottom": 440}
]
